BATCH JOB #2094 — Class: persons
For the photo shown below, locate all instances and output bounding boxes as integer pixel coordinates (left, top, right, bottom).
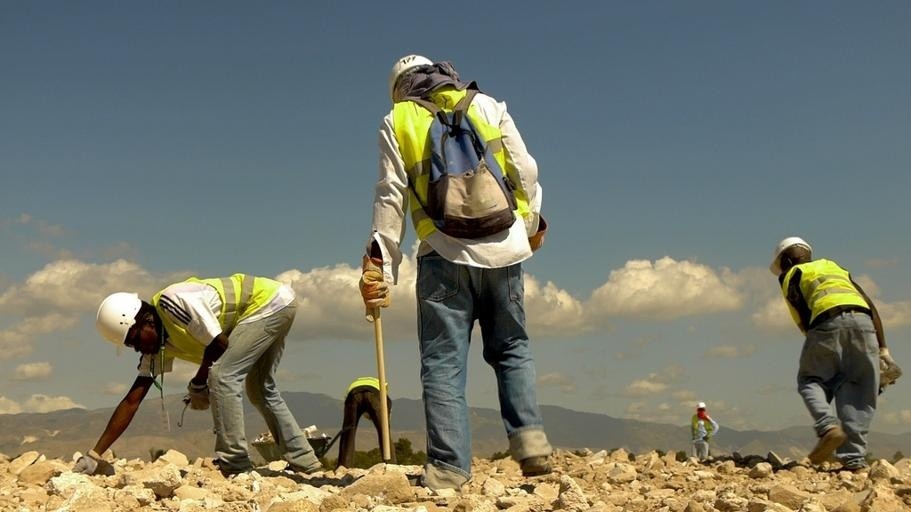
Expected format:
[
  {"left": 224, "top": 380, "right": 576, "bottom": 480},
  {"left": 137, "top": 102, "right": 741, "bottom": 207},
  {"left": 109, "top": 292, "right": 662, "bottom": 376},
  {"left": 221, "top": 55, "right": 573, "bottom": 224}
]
[
  {"left": 690, "top": 402, "right": 719, "bottom": 462},
  {"left": 337, "top": 376, "right": 397, "bottom": 469},
  {"left": 360, "top": 53, "right": 553, "bottom": 491},
  {"left": 770, "top": 237, "right": 897, "bottom": 475},
  {"left": 69, "top": 274, "right": 322, "bottom": 481}
]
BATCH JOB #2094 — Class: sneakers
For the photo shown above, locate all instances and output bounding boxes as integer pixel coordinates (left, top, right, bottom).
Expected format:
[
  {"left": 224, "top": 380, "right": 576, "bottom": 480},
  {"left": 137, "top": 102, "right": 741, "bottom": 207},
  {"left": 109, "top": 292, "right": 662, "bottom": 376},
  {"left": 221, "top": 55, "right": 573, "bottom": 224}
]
[
  {"left": 285, "top": 462, "right": 323, "bottom": 474},
  {"left": 808, "top": 428, "right": 869, "bottom": 473},
  {"left": 522, "top": 457, "right": 551, "bottom": 476}
]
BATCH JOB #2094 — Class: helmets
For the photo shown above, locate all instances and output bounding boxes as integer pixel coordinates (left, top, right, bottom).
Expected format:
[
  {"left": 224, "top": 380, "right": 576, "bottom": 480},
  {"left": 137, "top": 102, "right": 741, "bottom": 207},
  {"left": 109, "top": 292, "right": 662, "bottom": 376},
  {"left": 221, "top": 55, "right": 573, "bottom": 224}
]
[
  {"left": 770, "top": 237, "right": 813, "bottom": 275},
  {"left": 389, "top": 54, "right": 434, "bottom": 102},
  {"left": 96, "top": 292, "right": 142, "bottom": 354},
  {"left": 697, "top": 402, "right": 706, "bottom": 409}
]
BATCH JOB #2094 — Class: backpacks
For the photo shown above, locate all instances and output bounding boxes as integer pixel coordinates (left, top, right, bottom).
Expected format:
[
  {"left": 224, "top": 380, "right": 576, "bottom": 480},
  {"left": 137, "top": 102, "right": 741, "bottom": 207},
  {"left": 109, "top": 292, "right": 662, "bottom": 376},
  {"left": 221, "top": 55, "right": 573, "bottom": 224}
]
[{"left": 402, "top": 87, "right": 517, "bottom": 238}]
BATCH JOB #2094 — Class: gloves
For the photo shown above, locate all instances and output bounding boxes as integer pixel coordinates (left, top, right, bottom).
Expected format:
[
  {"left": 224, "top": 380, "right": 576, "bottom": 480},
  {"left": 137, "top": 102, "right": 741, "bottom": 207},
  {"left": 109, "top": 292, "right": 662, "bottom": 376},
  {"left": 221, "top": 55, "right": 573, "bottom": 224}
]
[
  {"left": 359, "top": 255, "right": 390, "bottom": 321},
  {"left": 188, "top": 382, "right": 211, "bottom": 410}
]
